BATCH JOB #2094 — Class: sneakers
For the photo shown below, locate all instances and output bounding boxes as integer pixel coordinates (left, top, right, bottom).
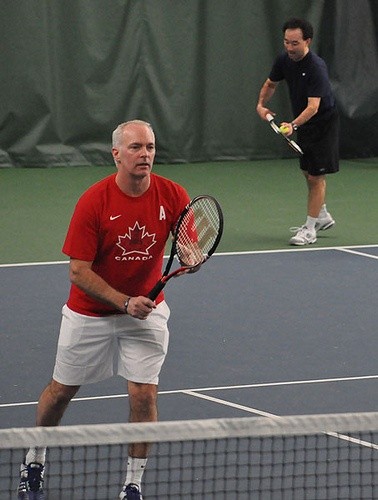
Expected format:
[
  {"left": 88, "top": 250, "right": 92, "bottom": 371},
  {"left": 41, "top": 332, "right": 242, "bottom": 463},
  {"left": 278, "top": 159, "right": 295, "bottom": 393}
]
[
  {"left": 118, "top": 483, "right": 143, "bottom": 500},
  {"left": 16, "top": 455, "right": 47, "bottom": 500},
  {"left": 315, "top": 212, "right": 335, "bottom": 232},
  {"left": 288, "top": 225, "right": 318, "bottom": 246}
]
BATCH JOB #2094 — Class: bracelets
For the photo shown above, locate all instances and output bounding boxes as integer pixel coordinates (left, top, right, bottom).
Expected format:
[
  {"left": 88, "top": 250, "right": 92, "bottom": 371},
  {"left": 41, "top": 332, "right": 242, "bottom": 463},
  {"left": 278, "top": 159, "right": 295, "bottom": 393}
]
[
  {"left": 291, "top": 122, "right": 297, "bottom": 131},
  {"left": 124, "top": 297, "right": 131, "bottom": 314}
]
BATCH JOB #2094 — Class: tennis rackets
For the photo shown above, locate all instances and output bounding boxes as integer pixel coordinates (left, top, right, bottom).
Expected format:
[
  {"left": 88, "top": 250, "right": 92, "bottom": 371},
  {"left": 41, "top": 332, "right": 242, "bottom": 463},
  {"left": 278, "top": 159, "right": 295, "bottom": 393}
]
[
  {"left": 269, "top": 114, "right": 305, "bottom": 156},
  {"left": 146, "top": 194, "right": 226, "bottom": 313}
]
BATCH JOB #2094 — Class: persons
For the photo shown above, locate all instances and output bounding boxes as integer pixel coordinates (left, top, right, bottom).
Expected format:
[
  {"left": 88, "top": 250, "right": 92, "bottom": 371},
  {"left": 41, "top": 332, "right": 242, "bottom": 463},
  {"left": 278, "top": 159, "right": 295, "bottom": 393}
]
[
  {"left": 16, "top": 120, "right": 203, "bottom": 500},
  {"left": 257, "top": 18, "right": 339, "bottom": 245}
]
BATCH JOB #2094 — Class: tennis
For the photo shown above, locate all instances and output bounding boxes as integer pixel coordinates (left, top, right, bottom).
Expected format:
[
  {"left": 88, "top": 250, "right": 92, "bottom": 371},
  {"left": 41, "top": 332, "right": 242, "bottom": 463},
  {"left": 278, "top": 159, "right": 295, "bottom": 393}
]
[{"left": 279, "top": 123, "right": 290, "bottom": 135}]
